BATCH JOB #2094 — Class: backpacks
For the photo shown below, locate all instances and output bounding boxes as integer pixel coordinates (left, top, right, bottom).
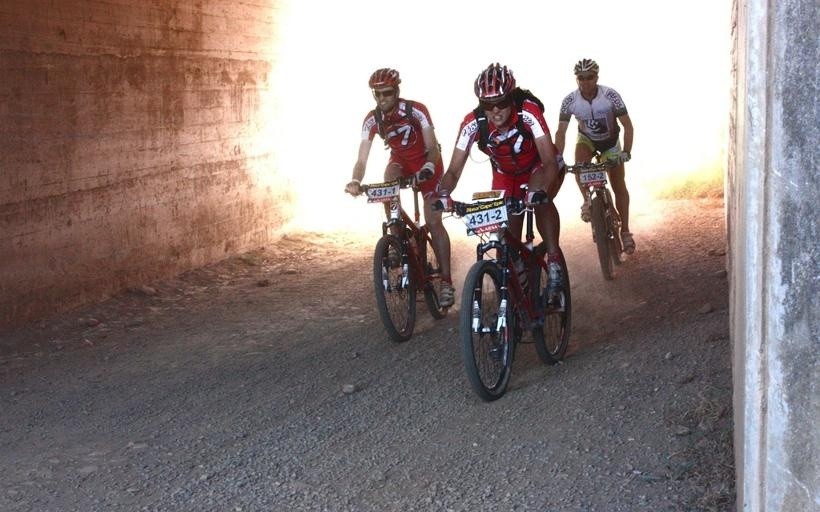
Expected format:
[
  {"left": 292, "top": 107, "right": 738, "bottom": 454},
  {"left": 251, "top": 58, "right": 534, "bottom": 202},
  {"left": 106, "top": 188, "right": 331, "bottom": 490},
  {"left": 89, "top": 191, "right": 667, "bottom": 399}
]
[{"left": 512, "top": 87, "right": 544, "bottom": 141}]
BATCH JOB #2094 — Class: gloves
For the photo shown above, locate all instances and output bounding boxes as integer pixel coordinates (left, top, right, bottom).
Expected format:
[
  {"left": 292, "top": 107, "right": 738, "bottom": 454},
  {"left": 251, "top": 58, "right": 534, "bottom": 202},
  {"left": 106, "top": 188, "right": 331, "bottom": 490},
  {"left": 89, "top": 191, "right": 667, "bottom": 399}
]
[
  {"left": 522, "top": 187, "right": 549, "bottom": 209},
  {"left": 416, "top": 162, "right": 437, "bottom": 185},
  {"left": 433, "top": 188, "right": 455, "bottom": 214},
  {"left": 616, "top": 149, "right": 632, "bottom": 166}
]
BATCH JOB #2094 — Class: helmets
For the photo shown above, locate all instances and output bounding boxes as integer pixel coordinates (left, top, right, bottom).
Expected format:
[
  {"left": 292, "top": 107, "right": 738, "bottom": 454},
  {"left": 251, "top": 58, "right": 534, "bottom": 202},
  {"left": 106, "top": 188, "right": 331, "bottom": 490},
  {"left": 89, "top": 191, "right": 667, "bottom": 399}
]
[
  {"left": 367, "top": 67, "right": 403, "bottom": 91},
  {"left": 474, "top": 63, "right": 517, "bottom": 106},
  {"left": 573, "top": 58, "right": 599, "bottom": 77}
]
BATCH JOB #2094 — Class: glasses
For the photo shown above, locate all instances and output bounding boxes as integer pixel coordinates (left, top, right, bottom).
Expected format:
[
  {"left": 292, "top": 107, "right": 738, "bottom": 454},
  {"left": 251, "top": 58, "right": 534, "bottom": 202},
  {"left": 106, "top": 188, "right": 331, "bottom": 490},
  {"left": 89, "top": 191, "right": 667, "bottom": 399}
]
[
  {"left": 371, "top": 89, "right": 396, "bottom": 97},
  {"left": 576, "top": 75, "right": 595, "bottom": 82},
  {"left": 478, "top": 99, "right": 510, "bottom": 111}
]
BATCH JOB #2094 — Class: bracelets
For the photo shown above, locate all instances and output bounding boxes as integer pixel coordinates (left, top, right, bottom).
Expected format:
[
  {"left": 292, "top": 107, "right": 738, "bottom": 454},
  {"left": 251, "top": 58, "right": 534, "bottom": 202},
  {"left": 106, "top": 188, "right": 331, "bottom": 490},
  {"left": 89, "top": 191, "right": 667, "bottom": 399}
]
[
  {"left": 351, "top": 179, "right": 360, "bottom": 184},
  {"left": 423, "top": 161, "right": 436, "bottom": 175}
]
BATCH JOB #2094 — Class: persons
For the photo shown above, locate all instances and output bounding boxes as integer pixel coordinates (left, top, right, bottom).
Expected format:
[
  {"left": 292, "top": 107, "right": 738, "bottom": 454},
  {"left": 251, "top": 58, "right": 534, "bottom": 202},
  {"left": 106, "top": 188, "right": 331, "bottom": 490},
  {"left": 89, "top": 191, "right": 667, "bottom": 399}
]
[
  {"left": 434, "top": 63, "right": 567, "bottom": 364},
  {"left": 344, "top": 67, "right": 456, "bottom": 308},
  {"left": 554, "top": 58, "right": 636, "bottom": 255}
]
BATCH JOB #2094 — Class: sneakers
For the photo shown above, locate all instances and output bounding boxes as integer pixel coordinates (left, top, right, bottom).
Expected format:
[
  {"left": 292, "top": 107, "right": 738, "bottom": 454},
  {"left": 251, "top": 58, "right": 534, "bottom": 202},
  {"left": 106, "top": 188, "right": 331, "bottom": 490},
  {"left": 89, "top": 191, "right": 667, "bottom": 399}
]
[
  {"left": 438, "top": 279, "right": 456, "bottom": 308},
  {"left": 544, "top": 252, "right": 567, "bottom": 291},
  {"left": 580, "top": 199, "right": 591, "bottom": 224},
  {"left": 619, "top": 229, "right": 636, "bottom": 256}
]
